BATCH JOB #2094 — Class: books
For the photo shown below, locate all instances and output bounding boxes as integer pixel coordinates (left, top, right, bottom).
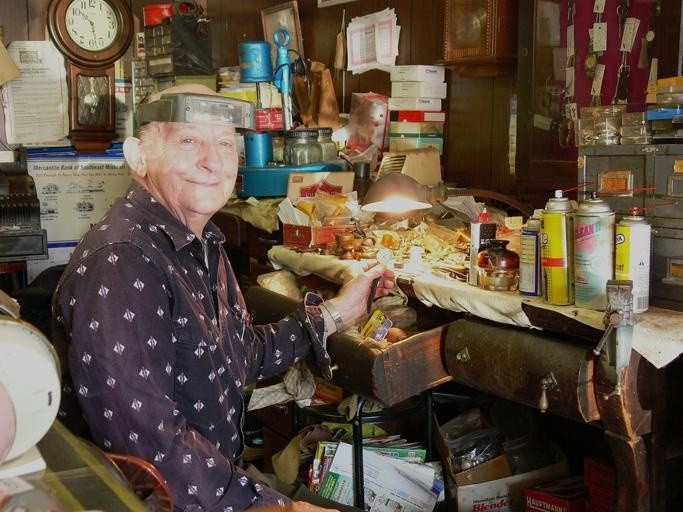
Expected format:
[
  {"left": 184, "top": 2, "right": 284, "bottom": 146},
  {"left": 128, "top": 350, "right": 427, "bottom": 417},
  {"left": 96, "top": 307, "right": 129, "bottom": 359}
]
[{"left": 310, "top": 433, "right": 442, "bottom": 512}]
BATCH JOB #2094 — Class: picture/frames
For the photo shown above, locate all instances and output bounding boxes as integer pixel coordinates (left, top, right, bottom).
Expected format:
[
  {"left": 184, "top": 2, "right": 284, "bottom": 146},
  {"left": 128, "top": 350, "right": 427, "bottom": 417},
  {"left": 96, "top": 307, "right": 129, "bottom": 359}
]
[{"left": 261, "top": 1, "right": 305, "bottom": 72}]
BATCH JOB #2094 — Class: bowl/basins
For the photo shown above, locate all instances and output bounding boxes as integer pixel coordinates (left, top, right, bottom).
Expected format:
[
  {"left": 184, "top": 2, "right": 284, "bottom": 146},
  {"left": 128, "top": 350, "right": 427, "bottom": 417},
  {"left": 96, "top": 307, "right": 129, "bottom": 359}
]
[{"left": 236, "top": 163, "right": 330, "bottom": 198}]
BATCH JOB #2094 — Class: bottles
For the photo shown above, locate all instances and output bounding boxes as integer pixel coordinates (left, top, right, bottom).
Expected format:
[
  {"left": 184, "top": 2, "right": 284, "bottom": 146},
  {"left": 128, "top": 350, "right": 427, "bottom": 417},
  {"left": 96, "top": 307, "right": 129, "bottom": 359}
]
[
  {"left": 352, "top": 163, "right": 370, "bottom": 196},
  {"left": 273, "top": 126, "right": 341, "bottom": 168},
  {"left": 478, "top": 187, "right": 651, "bottom": 316}
]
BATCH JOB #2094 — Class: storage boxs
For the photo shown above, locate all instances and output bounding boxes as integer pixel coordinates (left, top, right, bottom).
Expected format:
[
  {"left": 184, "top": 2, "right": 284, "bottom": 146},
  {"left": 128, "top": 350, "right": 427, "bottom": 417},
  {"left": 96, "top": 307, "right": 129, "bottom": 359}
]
[{"left": 434, "top": 399, "right": 572, "bottom": 512}]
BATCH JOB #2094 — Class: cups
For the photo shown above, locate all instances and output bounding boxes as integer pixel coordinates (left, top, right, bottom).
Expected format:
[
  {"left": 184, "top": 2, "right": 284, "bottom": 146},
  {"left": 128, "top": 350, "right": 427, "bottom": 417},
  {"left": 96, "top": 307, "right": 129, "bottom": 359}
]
[{"left": 244, "top": 133, "right": 272, "bottom": 166}]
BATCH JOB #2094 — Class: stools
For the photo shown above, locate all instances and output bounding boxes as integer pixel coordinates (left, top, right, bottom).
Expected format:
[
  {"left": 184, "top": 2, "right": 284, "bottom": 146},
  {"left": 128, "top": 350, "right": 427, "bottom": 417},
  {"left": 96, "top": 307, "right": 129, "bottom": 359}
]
[{"left": 291, "top": 391, "right": 434, "bottom": 512}]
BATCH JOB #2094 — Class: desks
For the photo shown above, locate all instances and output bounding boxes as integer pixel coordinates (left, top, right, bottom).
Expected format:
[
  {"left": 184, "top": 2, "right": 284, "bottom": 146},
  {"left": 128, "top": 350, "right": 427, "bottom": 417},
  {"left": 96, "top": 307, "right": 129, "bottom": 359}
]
[{"left": 245, "top": 243, "right": 683, "bottom": 490}]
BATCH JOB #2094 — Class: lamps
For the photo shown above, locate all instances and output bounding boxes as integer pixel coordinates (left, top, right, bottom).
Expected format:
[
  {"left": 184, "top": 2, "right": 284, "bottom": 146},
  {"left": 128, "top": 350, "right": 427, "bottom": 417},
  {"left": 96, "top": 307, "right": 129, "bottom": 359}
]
[{"left": 361, "top": 173, "right": 534, "bottom": 219}]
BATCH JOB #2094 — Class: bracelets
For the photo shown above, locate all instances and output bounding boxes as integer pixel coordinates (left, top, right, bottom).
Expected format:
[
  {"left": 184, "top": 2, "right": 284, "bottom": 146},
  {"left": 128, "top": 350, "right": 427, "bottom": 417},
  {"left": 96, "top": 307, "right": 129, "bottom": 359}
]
[{"left": 321, "top": 300, "right": 347, "bottom": 334}]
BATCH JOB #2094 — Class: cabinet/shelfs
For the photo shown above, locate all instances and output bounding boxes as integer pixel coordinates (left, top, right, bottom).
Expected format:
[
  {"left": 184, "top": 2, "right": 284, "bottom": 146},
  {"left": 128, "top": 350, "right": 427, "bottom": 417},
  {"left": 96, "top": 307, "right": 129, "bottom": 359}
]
[
  {"left": 578, "top": 143, "right": 682, "bottom": 314},
  {"left": 209, "top": 213, "right": 281, "bottom": 258}
]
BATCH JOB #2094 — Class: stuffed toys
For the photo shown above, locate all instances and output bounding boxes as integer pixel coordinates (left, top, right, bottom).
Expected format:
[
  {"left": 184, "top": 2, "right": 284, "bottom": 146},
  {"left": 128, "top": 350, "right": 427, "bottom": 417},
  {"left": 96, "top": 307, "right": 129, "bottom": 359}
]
[{"left": 357, "top": 104, "right": 389, "bottom": 148}]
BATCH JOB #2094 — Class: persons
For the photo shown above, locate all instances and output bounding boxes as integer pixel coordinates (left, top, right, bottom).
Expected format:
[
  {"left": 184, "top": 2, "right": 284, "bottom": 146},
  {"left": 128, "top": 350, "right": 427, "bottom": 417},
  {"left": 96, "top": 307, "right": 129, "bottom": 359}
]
[{"left": 48, "top": 85, "right": 397, "bottom": 512}]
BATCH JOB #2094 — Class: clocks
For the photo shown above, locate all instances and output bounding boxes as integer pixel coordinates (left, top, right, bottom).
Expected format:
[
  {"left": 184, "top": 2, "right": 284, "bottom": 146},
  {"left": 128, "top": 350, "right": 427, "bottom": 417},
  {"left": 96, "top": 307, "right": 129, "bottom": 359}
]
[
  {"left": 443, "top": 1, "right": 518, "bottom": 80},
  {"left": 46, "top": 0, "right": 134, "bottom": 154}
]
